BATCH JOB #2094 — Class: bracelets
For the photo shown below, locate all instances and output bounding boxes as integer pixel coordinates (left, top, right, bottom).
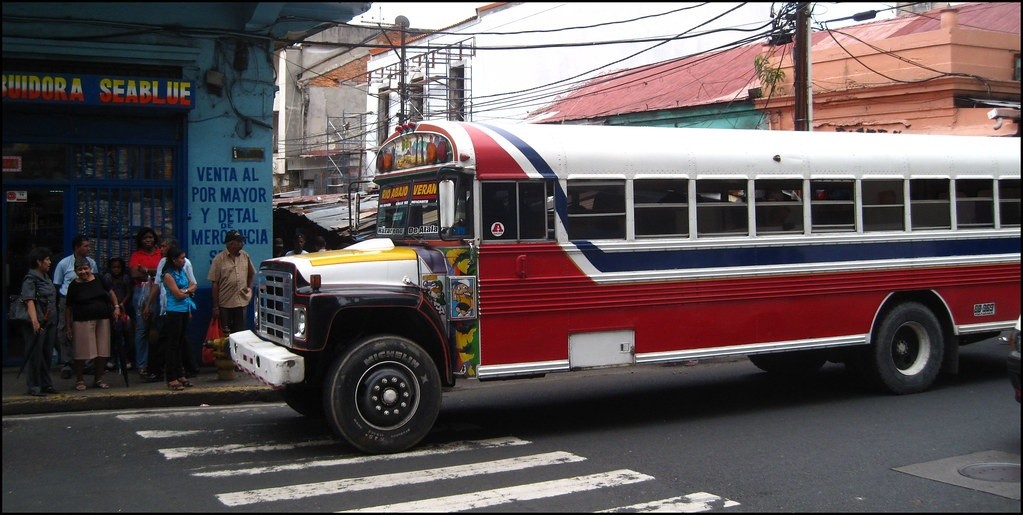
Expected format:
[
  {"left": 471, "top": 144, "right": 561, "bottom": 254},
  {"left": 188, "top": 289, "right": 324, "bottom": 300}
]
[
  {"left": 185, "top": 289, "right": 189, "bottom": 294},
  {"left": 114, "top": 305, "right": 120, "bottom": 308}
]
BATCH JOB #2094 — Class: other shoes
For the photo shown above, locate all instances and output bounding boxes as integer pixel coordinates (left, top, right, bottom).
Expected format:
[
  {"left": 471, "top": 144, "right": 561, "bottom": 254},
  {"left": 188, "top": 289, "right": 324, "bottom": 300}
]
[
  {"left": 144, "top": 371, "right": 164, "bottom": 383},
  {"left": 75, "top": 379, "right": 87, "bottom": 391},
  {"left": 29, "top": 385, "right": 56, "bottom": 395},
  {"left": 185, "top": 368, "right": 200, "bottom": 377},
  {"left": 95, "top": 380, "right": 110, "bottom": 389}
]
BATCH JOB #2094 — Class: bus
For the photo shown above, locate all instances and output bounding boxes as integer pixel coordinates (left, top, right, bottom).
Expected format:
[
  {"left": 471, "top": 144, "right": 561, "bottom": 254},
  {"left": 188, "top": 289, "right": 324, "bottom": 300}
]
[{"left": 225, "top": 119, "right": 1022, "bottom": 453}]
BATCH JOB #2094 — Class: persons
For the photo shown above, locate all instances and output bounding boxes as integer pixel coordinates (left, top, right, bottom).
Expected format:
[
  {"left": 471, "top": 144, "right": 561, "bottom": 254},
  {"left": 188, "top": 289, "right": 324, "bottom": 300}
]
[
  {"left": 53, "top": 235, "right": 98, "bottom": 380},
  {"left": 314, "top": 236, "right": 328, "bottom": 252},
  {"left": 141, "top": 239, "right": 200, "bottom": 382},
  {"left": 160, "top": 247, "right": 195, "bottom": 391},
  {"left": 128, "top": 228, "right": 164, "bottom": 375},
  {"left": 285, "top": 233, "right": 309, "bottom": 256},
  {"left": 273, "top": 238, "right": 286, "bottom": 258},
  {"left": 65, "top": 259, "right": 121, "bottom": 391},
  {"left": 101, "top": 257, "right": 133, "bottom": 370},
  {"left": 19, "top": 247, "right": 58, "bottom": 395},
  {"left": 206, "top": 230, "right": 255, "bottom": 373}
]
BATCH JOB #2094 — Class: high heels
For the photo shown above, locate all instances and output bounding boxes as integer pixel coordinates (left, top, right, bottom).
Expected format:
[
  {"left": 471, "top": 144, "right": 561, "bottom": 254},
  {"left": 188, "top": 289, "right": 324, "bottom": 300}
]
[{"left": 167, "top": 376, "right": 194, "bottom": 390}]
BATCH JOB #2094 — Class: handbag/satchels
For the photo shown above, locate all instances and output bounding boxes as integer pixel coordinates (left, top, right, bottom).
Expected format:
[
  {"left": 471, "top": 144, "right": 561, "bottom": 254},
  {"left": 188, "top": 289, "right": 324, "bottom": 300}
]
[{"left": 202, "top": 315, "right": 225, "bottom": 364}]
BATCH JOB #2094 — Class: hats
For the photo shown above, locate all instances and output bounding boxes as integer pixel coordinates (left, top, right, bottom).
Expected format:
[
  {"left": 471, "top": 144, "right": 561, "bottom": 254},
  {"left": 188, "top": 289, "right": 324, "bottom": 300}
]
[{"left": 224, "top": 229, "right": 247, "bottom": 243}]
